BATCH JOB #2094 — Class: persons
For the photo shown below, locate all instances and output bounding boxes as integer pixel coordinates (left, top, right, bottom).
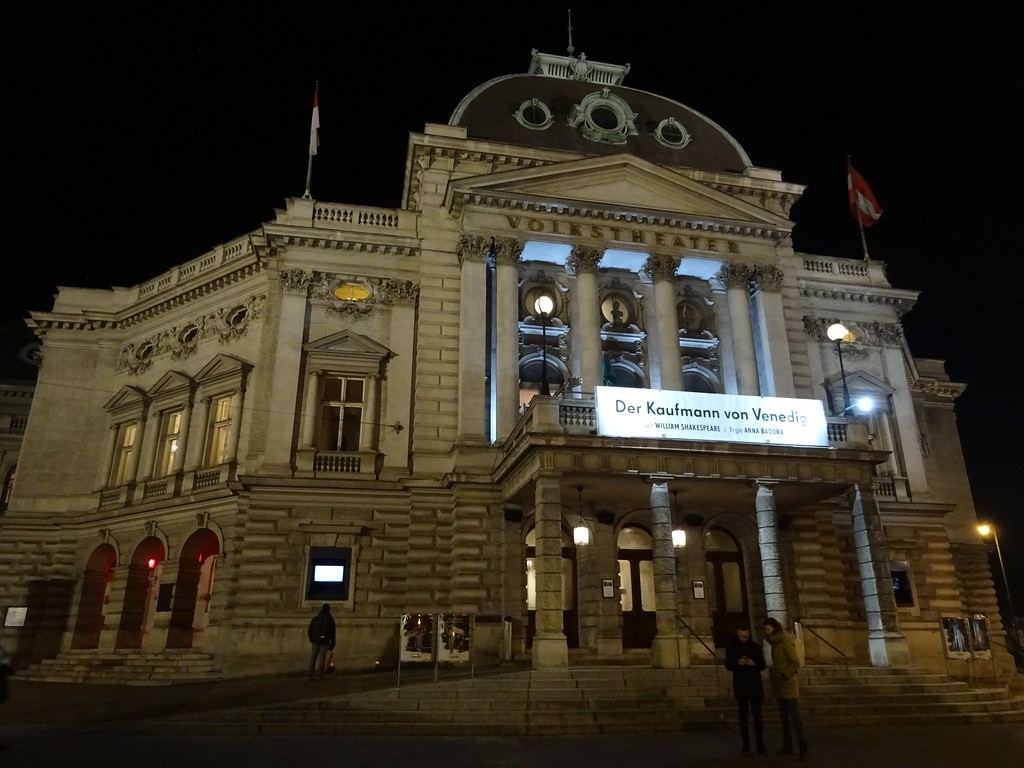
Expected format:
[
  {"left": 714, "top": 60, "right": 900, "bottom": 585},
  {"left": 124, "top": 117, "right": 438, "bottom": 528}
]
[
  {"left": 307, "top": 603, "right": 337, "bottom": 677},
  {"left": 760, "top": 618, "right": 808, "bottom": 753},
  {"left": 724, "top": 624, "right": 768, "bottom": 757}
]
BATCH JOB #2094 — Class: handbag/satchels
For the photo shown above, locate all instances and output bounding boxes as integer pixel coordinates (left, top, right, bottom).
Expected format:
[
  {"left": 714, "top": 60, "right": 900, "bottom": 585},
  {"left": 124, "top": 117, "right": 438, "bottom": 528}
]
[{"left": 325, "top": 652, "right": 336, "bottom": 674}]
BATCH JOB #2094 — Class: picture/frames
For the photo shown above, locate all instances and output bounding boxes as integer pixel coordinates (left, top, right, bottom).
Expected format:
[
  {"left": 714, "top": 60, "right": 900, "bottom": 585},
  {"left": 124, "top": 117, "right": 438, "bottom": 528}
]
[
  {"left": 693, "top": 580, "right": 705, "bottom": 599},
  {"left": 602, "top": 579, "right": 615, "bottom": 597}
]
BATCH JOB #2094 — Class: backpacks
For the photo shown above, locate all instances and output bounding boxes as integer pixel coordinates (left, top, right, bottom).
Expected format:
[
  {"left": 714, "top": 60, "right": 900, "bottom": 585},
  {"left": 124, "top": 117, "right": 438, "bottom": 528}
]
[{"left": 313, "top": 615, "right": 334, "bottom": 644}]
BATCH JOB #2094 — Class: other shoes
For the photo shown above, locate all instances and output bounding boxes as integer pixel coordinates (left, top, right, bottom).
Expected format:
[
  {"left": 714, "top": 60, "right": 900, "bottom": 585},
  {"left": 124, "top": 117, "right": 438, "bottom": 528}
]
[
  {"left": 742, "top": 744, "right": 752, "bottom": 754},
  {"left": 756, "top": 745, "right": 769, "bottom": 756}
]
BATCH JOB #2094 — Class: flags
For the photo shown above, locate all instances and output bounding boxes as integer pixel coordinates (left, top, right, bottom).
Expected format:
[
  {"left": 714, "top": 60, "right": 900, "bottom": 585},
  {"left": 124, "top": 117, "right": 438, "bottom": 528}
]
[
  {"left": 312, "top": 91, "right": 320, "bottom": 156},
  {"left": 848, "top": 168, "right": 883, "bottom": 227}
]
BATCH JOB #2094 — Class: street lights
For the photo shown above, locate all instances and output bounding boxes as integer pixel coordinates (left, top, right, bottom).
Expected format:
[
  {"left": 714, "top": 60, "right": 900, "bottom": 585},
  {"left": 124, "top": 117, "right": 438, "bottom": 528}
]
[{"left": 976, "top": 524, "right": 1016, "bottom": 625}]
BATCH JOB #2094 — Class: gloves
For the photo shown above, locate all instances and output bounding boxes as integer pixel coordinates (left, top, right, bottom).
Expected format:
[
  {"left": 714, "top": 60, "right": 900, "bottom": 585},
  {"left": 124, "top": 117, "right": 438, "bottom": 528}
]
[{"left": 328, "top": 644, "right": 334, "bottom": 651}]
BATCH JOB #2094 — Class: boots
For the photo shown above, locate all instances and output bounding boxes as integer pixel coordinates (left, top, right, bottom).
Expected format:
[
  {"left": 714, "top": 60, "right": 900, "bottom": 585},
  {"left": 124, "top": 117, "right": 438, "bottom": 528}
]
[
  {"left": 775, "top": 737, "right": 794, "bottom": 756},
  {"left": 797, "top": 740, "right": 810, "bottom": 761}
]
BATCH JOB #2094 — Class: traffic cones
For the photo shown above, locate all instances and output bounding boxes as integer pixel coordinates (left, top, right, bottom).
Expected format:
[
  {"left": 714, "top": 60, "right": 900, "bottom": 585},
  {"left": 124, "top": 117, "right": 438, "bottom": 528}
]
[{"left": 328, "top": 653, "right": 334, "bottom": 667}]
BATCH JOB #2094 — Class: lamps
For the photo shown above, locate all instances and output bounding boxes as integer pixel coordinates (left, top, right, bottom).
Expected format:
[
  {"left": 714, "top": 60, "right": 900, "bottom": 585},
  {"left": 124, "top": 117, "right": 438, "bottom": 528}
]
[
  {"left": 575, "top": 486, "right": 589, "bottom": 545},
  {"left": 672, "top": 489, "right": 687, "bottom": 548}
]
